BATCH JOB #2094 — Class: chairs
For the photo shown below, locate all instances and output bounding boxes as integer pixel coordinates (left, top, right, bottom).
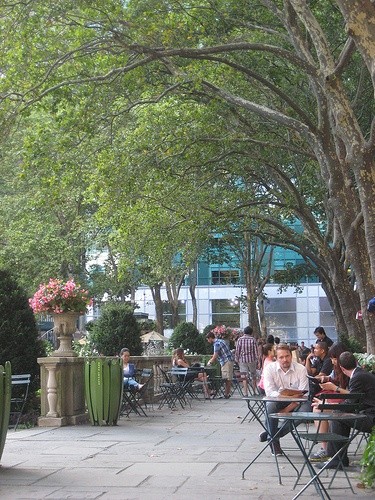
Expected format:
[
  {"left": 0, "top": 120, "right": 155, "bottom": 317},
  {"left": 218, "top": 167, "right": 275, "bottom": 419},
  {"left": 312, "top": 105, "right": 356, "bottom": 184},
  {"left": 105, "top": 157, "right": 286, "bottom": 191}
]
[
  {"left": 210, "top": 375, "right": 258, "bottom": 398},
  {"left": 10, "top": 375, "right": 32, "bottom": 431},
  {"left": 296, "top": 394, "right": 364, "bottom": 496},
  {"left": 156, "top": 362, "right": 204, "bottom": 410},
  {"left": 121, "top": 369, "right": 153, "bottom": 418}
]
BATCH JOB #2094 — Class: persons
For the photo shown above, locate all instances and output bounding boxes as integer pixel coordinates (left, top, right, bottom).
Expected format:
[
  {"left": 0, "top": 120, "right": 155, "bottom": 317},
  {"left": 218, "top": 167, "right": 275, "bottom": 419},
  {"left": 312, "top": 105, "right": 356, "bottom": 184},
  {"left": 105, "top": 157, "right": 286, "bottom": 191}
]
[
  {"left": 235, "top": 327, "right": 261, "bottom": 396},
  {"left": 259, "top": 343, "right": 310, "bottom": 457},
  {"left": 257, "top": 343, "right": 276, "bottom": 395},
  {"left": 172, "top": 347, "right": 210, "bottom": 399},
  {"left": 206, "top": 332, "right": 234, "bottom": 399},
  {"left": 305, "top": 342, "right": 333, "bottom": 398},
  {"left": 313, "top": 327, "right": 334, "bottom": 349},
  {"left": 315, "top": 351, "right": 375, "bottom": 469},
  {"left": 274, "top": 337, "right": 280, "bottom": 352},
  {"left": 119, "top": 348, "right": 147, "bottom": 390},
  {"left": 291, "top": 342, "right": 319, "bottom": 365},
  {"left": 260, "top": 334, "right": 275, "bottom": 369},
  {"left": 307, "top": 344, "right": 363, "bottom": 462}
]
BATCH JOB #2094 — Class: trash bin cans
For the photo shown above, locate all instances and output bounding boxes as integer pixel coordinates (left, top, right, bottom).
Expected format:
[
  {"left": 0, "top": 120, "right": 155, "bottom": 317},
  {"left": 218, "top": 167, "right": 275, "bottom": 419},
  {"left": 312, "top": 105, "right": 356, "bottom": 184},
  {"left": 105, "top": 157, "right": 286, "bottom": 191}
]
[
  {"left": 205, "top": 356, "right": 221, "bottom": 378},
  {"left": 84, "top": 357, "right": 123, "bottom": 427},
  {"left": 0, "top": 361, "right": 12, "bottom": 462}
]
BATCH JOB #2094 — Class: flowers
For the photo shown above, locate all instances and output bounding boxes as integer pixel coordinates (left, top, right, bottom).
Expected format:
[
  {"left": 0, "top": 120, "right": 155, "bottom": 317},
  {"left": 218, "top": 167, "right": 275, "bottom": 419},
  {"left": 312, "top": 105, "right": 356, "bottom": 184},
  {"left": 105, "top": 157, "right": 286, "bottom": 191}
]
[{"left": 29, "top": 276, "right": 93, "bottom": 315}]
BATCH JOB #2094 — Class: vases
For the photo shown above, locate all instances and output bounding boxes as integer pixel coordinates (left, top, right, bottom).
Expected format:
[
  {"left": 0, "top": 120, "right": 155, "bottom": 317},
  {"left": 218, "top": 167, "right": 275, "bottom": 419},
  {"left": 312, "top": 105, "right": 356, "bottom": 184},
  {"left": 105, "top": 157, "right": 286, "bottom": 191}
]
[{"left": 46, "top": 313, "right": 82, "bottom": 357}]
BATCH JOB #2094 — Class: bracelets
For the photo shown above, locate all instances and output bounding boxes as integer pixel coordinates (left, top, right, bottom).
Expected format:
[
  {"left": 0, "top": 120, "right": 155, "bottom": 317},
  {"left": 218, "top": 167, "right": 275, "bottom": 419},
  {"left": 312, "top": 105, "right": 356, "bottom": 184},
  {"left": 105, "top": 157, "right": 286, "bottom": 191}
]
[
  {"left": 336, "top": 386, "right": 340, "bottom": 392},
  {"left": 321, "top": 376, "right": 325, "bottom": 383}
]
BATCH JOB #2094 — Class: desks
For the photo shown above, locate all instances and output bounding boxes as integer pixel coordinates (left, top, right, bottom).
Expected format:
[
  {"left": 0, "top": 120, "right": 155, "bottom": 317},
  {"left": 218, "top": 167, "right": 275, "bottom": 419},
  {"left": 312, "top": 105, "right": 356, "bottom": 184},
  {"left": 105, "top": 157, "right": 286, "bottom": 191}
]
[
  {"left": 269, "top": 413, "right": 367, "bottom": 499},
  {"left": 189, "top": 367, "right": 218, "bottom": 400},
  {"left": 242, "top": 396, "right": 307, "bottom": 479}
]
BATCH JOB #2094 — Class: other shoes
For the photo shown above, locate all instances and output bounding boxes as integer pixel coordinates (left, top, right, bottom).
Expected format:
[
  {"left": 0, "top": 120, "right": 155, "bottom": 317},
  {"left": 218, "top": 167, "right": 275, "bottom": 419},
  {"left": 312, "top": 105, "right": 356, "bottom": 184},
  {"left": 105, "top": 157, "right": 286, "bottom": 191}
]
[
  {"left": 315, "top": 458, "right": 349, "bottom": 469},
  {"left": 219, "top": 394, "right": 231, "bottom": 399}
]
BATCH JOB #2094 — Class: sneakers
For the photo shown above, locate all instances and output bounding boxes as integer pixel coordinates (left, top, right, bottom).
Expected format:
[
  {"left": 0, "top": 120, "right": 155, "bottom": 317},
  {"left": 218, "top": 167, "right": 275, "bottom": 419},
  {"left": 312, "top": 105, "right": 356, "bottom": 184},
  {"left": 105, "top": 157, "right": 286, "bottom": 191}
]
[
  {"left": 310, "top": 448, "right": 326, "bottom": 459},
  {"left": 259, "top": 431, "right": 268, "bottom": 442},
  {"left": 268, "top": 438, "right": 284, "bottom": 456}
]
